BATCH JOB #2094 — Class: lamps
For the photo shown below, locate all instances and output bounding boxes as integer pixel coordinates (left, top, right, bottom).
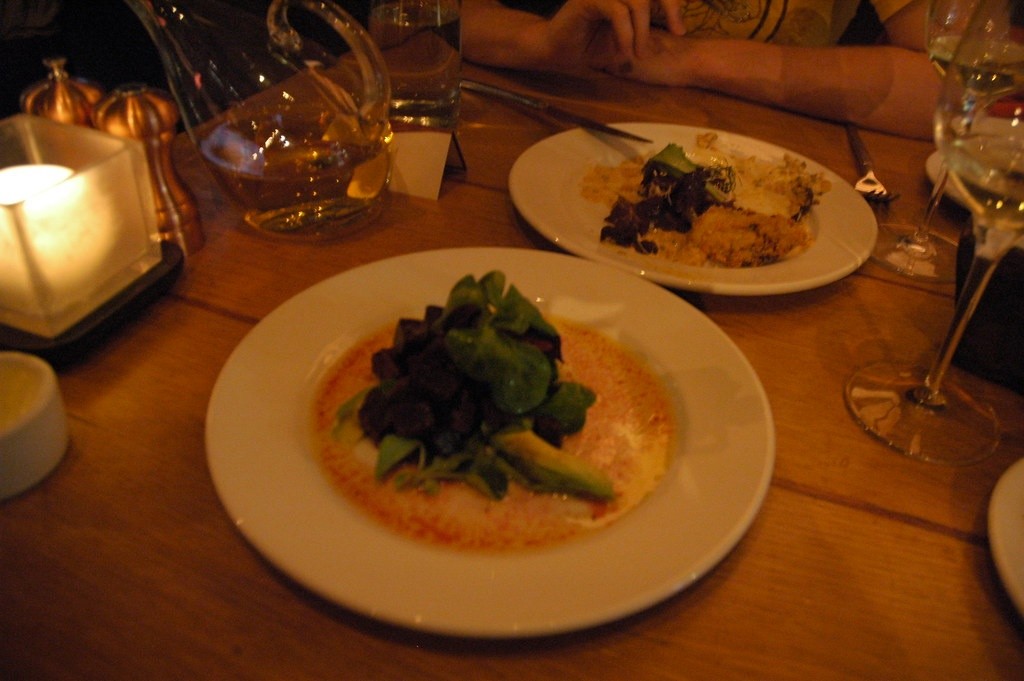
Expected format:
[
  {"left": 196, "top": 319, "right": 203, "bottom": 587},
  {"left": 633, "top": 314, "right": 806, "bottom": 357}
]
[{"left": 0, "top": 114, "right": 183, "bottom": 369}]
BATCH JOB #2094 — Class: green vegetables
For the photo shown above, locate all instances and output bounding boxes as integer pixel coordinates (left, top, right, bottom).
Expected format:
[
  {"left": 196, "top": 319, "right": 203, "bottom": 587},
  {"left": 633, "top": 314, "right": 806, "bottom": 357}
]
[
  {"left": 330, "top": 270, "right": 616, "bottom": 504},
  {"left": 597, "top": 143, "right": 737, "bottom": 254}
]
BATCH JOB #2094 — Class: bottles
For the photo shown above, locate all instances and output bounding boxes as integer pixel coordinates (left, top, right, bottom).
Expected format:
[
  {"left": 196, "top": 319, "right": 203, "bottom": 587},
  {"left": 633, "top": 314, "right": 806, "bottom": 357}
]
[{"left": 20, "top": 55, "right": 205, "bottom": 257}]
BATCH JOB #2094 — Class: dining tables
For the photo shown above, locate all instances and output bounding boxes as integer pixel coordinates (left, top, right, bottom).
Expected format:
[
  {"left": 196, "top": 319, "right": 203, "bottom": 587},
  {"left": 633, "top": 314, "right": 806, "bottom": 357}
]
[{"left": 0, "top": 51, "right": 1024, "bottom": 681}]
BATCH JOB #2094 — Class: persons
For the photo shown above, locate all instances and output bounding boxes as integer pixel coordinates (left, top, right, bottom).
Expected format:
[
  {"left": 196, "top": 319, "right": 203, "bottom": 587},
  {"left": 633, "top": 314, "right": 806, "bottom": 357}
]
[{"left": 458, "top": 0, "right": 1024, "bottom": 141}]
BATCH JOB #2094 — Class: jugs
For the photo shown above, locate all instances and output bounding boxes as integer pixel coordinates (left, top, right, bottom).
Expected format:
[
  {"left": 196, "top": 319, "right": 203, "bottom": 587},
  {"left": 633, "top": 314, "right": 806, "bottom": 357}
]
[{"left": 127, "top": 0, "right": 393, "bottom": 243}]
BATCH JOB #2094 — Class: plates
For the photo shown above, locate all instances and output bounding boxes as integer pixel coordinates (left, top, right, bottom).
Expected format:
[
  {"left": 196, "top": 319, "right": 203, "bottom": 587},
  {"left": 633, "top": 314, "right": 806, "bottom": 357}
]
[
  {"left": 508, "top": 122, "right": 879, "bottom": 296},
  {"left": 987, "top": 458, "right": 1024, "bottom": 628},
  {"left": 204, "top": 247, "right": 776, "bottom": 639}
]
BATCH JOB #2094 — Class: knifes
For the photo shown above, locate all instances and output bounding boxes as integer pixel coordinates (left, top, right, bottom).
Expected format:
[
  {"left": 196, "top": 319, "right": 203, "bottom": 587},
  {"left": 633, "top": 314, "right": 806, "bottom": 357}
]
[{"left": 460, "top": 79, "right": 652, "bottom": 144}]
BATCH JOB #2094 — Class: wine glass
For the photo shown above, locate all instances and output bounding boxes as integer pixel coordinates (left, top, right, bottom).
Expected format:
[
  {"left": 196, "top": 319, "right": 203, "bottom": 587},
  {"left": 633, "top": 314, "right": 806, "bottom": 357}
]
[
  {"left": 845, "top": 0, "right": 1024, "bottom": 464},
  {"left": 870, "top": 0, "right": 1024, "bottom": 282}
]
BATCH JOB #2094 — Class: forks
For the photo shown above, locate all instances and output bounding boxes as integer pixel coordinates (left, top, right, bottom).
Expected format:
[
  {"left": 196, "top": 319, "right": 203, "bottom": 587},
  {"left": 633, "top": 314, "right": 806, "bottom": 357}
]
[{"left": 844, "top": 120, "right": 902, "bottom": 204}]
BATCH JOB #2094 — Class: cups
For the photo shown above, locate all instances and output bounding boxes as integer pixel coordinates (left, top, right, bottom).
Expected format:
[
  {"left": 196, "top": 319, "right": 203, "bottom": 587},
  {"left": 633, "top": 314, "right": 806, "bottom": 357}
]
[
  {"left": 0, "top": 351, "right": 70, "bottom": 501},
  {"left": 368, "top": 0, "right": 461, "bottom": 127}
]
